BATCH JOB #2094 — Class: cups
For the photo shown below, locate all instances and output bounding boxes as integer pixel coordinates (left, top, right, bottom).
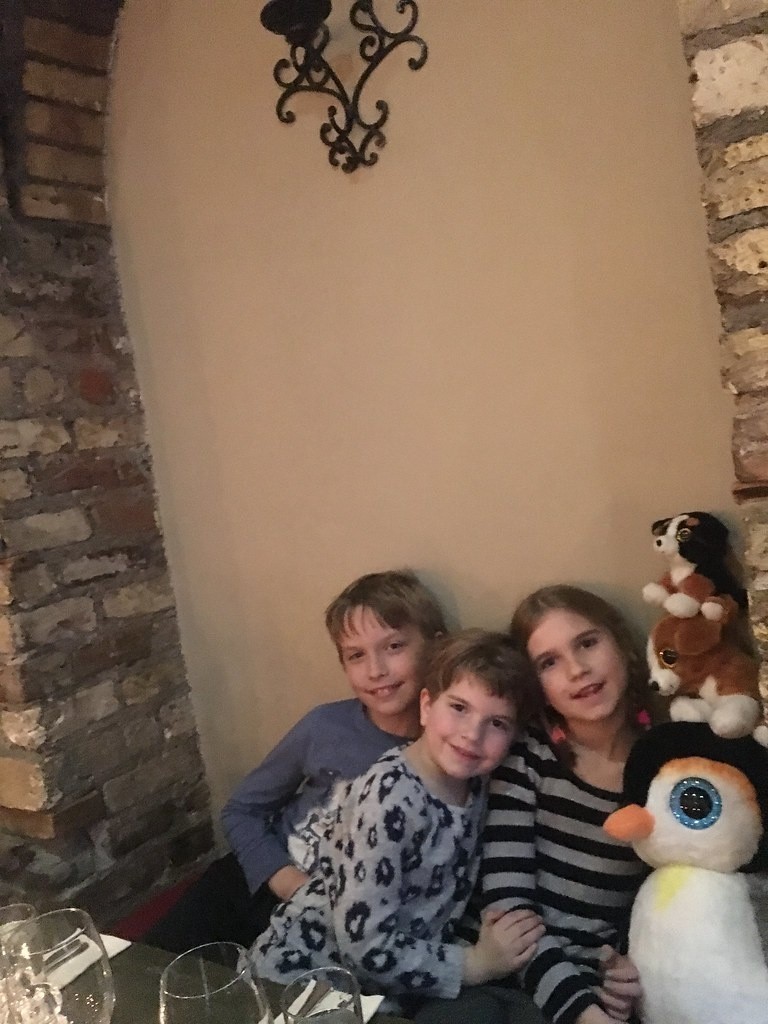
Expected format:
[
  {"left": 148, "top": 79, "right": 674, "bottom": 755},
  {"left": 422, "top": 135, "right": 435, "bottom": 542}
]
[
  {"left": 283, "top": 967, "right": 362, "bottom": 1022},
  {"left": 159, "top": 940, "right": 271, "bottom": 1024},
  {"left": 2, "top": 903, "right": 117, "bottom": 1024}
]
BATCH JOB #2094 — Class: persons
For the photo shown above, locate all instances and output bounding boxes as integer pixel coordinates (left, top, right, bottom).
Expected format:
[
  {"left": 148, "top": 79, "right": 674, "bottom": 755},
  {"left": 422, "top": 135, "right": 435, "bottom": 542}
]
[
  {"left": 236, "top": 626, "right": 549, "bottom": 1024},
  {"left": 101, "top": 564, "right": 449, "bottom": 1023},
  {"left": 484, "top": 583, "right": 649, "bottom": 1017}
]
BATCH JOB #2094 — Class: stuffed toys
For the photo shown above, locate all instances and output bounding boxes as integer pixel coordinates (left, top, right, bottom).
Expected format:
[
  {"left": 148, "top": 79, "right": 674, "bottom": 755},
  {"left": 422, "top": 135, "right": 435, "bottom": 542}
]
[
  {"left": 601, "top": 720, "right": 767, "bottom": 1024},
  {"left": 638, "top": 509, "right": 768, "bottom": 750}
]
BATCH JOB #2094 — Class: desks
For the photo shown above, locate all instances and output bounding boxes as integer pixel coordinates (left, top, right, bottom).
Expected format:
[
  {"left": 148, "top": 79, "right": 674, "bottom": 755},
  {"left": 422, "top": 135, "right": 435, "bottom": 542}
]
[{"left": 0, "top": 907, "right": 408, "bottom": 1024}]
